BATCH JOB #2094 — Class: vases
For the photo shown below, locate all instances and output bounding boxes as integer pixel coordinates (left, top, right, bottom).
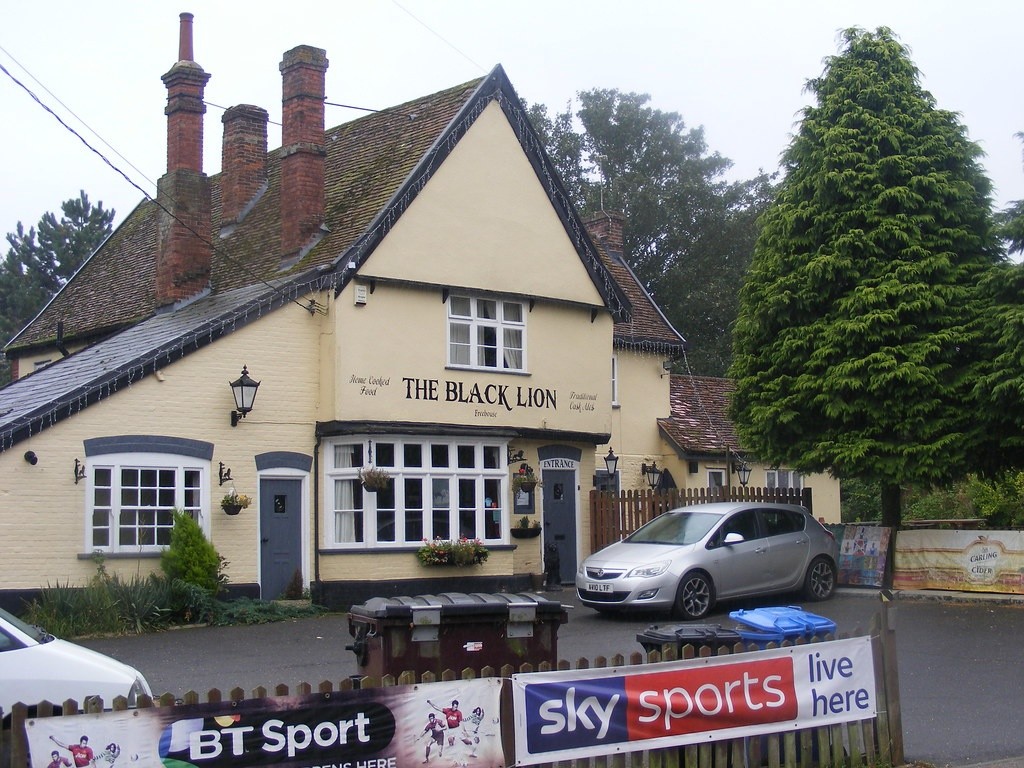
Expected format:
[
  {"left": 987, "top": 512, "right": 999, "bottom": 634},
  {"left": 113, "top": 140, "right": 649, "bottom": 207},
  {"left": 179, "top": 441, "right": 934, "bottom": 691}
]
[
  {"left": 519, "top": 481, "right": 538, "bottom": 494},
  {"left": 220, "top": 504, "right": 243, "bottom": 515}
]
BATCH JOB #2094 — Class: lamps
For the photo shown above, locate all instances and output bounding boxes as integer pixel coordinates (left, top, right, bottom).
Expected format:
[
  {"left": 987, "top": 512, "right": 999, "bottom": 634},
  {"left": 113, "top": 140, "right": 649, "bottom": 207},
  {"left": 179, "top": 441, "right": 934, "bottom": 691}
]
[
  {"left": 593, "top": 447, "right": 620, "bottom": 486},
  {"left": 641, "top": 460, "right": 663, "bottom": 489},
  {"left": 229, "top": 362, "right": 262, "bottom": 427},
  {"left": 730, "top": 459, "right": 752, "bottom": 487}
]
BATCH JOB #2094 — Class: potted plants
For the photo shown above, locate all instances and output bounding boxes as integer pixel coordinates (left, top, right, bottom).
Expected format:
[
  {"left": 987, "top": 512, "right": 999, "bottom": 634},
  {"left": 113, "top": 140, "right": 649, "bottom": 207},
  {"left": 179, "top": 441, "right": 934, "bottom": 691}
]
[{"left": 357, "top": 467, "right": 390, "bottom": 493}]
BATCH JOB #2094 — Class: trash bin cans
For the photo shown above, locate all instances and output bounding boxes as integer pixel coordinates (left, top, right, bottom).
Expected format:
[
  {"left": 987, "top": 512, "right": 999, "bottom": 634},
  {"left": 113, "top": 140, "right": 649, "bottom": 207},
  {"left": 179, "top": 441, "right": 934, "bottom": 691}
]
[
  {"left": 727, "top": 603, "right": 839, "bottom": 764},
  {"left": 637, "top": 623, "right": 737, "bottom": 767},
  {"left": 344, "top": 591, "right": 571, "bottom": 689}
]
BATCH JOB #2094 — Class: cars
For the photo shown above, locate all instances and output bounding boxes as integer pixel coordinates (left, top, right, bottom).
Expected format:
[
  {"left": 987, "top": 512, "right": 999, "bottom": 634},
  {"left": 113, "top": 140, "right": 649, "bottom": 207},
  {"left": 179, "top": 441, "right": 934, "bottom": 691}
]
[
  {"left": 0, "top": 605, "right": 153, "bottom": 748},
  {"left": 574, "top": 502, "right": 840, "bottom": 624}
]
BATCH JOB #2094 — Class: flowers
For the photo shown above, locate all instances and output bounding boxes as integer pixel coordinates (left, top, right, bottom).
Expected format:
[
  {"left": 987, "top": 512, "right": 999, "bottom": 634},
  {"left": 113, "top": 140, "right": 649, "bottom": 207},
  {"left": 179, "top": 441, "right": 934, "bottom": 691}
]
[
  {"left": 512, "top": 464, "right": 546, "bottom": 499},
  {"left": 220, "top": 490, "right": 253, "bottom": 510}
]
